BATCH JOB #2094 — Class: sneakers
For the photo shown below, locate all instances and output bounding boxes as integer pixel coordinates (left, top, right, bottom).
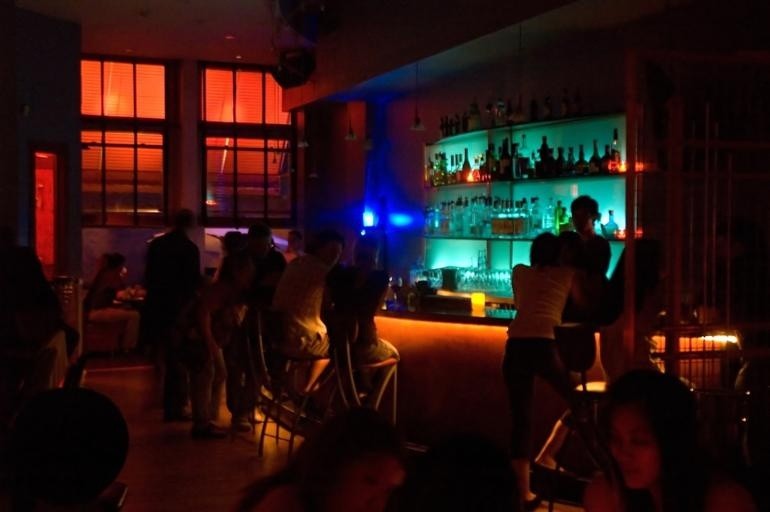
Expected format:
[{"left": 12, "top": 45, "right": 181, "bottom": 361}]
[
  {"left": 522, "top": 490, "right": 543, "bottom": 512},
  {"left": 164, "top": 409, "right": 263, "bottom": 438}
]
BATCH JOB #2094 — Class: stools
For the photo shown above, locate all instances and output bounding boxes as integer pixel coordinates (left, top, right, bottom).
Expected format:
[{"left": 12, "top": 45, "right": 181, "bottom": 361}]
[{"left": 82, "top": 320, "right": 118, "bottom": 354}]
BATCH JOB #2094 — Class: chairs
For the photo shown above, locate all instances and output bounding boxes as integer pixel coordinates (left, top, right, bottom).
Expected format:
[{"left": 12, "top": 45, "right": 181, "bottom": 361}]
[
  {"left": 249, "top": 304, "right": 400, "bottom": 462},
  {"left": 555, "top": 327, "right": 608, "bottom": 424}
]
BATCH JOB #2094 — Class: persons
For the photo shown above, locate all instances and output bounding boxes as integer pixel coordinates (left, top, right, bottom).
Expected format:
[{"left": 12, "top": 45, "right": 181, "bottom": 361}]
[
  {"left": 1, "top": 198, "right": 667, "bottom": 512},
  {"left": 582, "top": 368, "right": 759, "bottom": 512},
  {"left": 698, "top": 212, "right": 770, "bottom": 394}
]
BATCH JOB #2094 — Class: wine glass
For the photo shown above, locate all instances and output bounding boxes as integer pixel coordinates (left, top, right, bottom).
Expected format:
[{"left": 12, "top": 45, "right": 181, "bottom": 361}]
[{"left": 412, "top": 244, "right": 516, "bottom": 298}]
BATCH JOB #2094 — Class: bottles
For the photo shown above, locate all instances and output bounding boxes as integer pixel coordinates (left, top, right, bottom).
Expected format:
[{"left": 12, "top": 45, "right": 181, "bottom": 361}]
[
  {"left": 427, "top": 87, "right": 626, "bottom": 240},
  {"left": 380, "top": 274, "right": 409, "bottom": 315}
]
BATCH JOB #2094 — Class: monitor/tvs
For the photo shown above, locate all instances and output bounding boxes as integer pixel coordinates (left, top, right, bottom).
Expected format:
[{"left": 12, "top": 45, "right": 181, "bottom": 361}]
[{"left": 611, "top": 239, "right": 668, "bottom": 285}]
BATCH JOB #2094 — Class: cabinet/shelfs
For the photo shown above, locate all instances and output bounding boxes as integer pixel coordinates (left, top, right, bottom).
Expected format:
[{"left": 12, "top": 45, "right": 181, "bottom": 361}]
[{"left": 423, "top": 114, "right": 626, "bottom": 240}]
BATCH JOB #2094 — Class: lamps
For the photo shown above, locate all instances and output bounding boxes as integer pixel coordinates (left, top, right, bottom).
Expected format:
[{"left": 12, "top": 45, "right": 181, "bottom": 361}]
[
  {"left": 411, "top": 64, "right": 426, "bottom": 131},
  {"left": 344, "top": 119, "right": 356, "bottom": 140},
  {"left": 510, "top": 24, "right": 527, "bottom": 121}
]
